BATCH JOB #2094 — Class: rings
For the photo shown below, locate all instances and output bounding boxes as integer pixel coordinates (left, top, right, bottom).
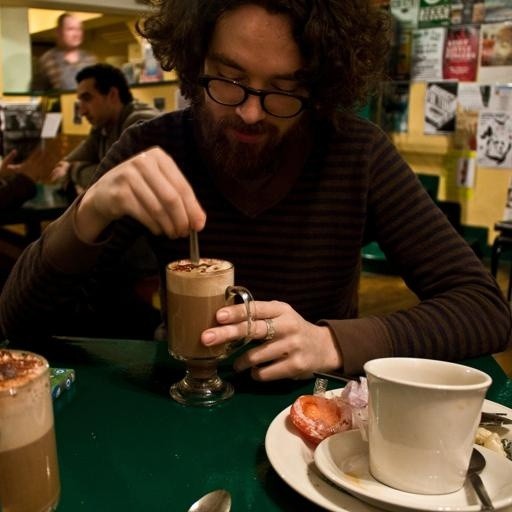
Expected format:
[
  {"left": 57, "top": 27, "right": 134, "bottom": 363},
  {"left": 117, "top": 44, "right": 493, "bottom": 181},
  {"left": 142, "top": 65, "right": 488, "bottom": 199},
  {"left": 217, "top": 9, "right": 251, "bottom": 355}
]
[{"left": 262, "top": 318, "right": 275, "bottom": 341}]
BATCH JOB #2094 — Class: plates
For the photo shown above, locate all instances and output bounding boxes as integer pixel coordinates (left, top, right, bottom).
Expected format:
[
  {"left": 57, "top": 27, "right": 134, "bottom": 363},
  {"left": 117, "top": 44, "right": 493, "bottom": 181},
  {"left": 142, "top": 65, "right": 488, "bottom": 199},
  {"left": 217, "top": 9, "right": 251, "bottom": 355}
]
[{"left": 265, "top": 387, "right": 512, "bottom": 511}]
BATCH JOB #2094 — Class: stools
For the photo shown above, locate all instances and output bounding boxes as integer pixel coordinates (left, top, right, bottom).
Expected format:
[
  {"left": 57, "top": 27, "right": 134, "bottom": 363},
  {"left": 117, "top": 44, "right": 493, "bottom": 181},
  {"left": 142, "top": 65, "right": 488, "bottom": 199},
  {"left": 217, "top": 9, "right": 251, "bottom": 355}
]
[{"left": 490, "top": 220, "right": 512, "bottom": 303}]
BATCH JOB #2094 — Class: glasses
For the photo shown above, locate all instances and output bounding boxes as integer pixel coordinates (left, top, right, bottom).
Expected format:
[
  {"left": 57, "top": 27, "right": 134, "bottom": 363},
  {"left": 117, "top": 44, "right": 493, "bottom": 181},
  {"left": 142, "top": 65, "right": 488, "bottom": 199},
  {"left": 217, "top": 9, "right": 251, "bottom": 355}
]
[{"left": 197, "top": 62, "right": 313, "bottom": 118}]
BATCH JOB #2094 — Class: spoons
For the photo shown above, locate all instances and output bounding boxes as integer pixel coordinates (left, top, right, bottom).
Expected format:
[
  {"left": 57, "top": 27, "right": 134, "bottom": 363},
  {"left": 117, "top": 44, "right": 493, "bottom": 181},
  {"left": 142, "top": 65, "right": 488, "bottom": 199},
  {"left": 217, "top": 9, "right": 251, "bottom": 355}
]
[{"left": 188, "top": 489, "right": 233, "bottom": 512}]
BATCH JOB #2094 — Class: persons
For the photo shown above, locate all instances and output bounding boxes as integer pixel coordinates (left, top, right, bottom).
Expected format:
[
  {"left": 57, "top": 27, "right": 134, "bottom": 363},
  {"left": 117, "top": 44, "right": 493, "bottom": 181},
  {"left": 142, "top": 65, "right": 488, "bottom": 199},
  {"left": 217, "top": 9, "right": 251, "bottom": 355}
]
[
  {"left": 48, "top": 64, "right": 162, "bottom": 341},
  {"left": 1, "top": 146, "right": 41, "bottom": 291},
  {"left": 2, "top": 0, "right": 512, "bottom": 383},
  {"left": 29, "top": 15, "right": 105, "bottom": 95}
]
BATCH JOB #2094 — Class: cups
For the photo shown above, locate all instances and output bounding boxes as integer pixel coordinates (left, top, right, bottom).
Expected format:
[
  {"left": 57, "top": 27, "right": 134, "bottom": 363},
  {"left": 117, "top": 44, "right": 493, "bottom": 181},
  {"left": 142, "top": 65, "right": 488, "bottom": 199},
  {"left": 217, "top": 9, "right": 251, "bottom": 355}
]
[
  {"left": 363, "top": 357, "right": 492, "bottom": 495},
  {"left": 0, "top": 348, "right": 61, "bottom": 511}
]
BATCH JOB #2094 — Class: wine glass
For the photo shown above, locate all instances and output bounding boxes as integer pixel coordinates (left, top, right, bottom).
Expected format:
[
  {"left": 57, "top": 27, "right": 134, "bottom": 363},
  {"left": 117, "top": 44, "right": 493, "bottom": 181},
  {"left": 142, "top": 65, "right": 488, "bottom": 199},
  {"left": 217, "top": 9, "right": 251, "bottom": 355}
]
[{"left": 167, "top": 258, "right": 255, "bottom": 406}]
